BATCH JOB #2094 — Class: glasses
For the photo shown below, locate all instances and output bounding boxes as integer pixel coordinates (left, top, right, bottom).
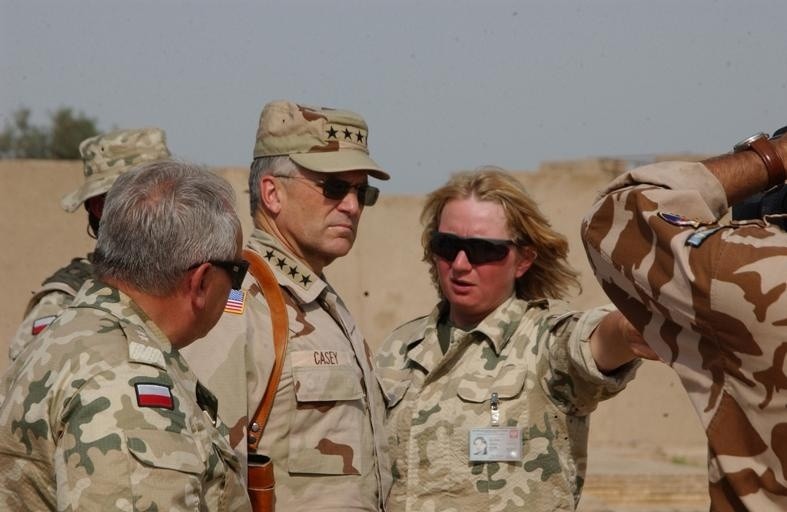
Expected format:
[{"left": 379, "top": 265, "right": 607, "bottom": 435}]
[
  {"left": 187, "top": 260, "right": 249, "bottom": 290},
  {"left": 430, "top": 233, "right": 513, "bottom": 264},
  {"left": 275, "top": 175, "right": 380, "bottom": 206}
]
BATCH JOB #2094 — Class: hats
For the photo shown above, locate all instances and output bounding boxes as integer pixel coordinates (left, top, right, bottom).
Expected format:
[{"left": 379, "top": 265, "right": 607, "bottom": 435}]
[
  {"left": 253, "top": 100, "right": 390, "bottom": 180},
  {"left": 59, "top": 127, "right": 172, "bottom": 213}
]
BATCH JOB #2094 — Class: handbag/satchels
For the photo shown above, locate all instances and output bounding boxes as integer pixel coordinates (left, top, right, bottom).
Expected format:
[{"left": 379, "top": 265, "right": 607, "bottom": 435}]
[{"left": 247, "top": 454, "right": 276, "bottom": 512}]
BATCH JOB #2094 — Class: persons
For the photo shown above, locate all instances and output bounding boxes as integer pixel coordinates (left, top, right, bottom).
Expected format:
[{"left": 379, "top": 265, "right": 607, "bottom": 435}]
[
  {"left": 372, "top": 166, "right": 663, "bottom": 512},
  {"left": 178, "top": 102, "right": 393, "bottom": 512},
  {"left": 1, "top": 159, "right": 252, "bottom": 512},
  {"left": 9, "top": 126, "right": 169, "bottom": 360},
  {"left": 580, "top": 128, "right": 786, "bottom": 512}
]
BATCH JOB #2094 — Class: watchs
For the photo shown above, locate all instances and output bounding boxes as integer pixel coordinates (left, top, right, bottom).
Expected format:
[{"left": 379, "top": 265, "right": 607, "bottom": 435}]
[{"left": 733, "top": 131, "right": 786, "bottom": 188}]
